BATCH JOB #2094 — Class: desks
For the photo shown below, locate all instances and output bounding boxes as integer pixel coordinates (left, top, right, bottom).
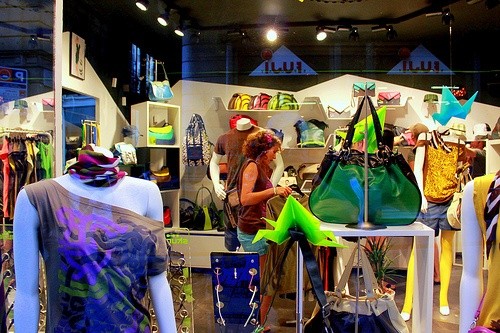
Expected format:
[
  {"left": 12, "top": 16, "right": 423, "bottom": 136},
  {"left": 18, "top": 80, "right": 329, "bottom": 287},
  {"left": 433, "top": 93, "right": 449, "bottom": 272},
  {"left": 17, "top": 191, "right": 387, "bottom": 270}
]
[{"left": 294, "top": 217, "right": 435, "bottom": 333}]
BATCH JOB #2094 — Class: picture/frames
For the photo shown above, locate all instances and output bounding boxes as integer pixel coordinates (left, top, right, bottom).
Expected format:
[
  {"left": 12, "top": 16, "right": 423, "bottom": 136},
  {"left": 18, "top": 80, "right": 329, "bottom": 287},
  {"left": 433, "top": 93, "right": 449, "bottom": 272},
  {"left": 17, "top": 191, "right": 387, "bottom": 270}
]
[{"left": 69, "top": 31, "right": 86, "bottom": 80}]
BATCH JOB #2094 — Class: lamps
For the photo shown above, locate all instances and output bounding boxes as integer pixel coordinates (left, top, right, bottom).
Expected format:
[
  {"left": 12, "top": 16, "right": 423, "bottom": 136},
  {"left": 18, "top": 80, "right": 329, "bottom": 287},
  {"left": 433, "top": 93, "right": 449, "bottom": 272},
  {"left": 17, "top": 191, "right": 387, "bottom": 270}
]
[
  {"left": 136, "top": 0, "right": 150, "bottom": 11},
  {"left": 174, "top": 17, "right": 187, "bottom": 36},
  {"left": 156, "top": 7, "right": 172, "bottom": 26},
  {"left": 190, "top": 1, "right": 496, "bottom": 43}
]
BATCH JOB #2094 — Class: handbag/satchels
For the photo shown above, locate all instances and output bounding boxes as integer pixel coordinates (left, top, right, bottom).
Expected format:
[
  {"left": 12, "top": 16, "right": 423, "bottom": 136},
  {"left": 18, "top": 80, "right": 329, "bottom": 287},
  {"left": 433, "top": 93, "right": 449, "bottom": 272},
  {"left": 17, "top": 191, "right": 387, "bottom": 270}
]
[
  {"left": 178, "top": 187, "right": 222, "bottom": 231},
  {"left": 308, "top": 96, "right": 422, "bottom": 226},
  {"left": 447, "top": 162, "right": 475, "bottom": 229},
  {"left": 303, "top": 244, "right": 409, "bottom": 333},
  {"left": 224, "top": 222, "right": 241, "bottom": 251},
  {"left": 147, "top": 77, "right": 173, "bottom": 100},
  {"left": 467, "top": 324, "right": 495, "bottom": 333},
  {"left": 295, "top": 119, "right": 328, "bottom": 148},
  {"left": 377, "top": 92, "right": 402, "bottom": 105},
  {"left": 149, "top": 123, "right": 176, "bottom": 146},
  {"left": 111, "top": 140, "right": 138, "bottom": 165},
  {"left": 353, "top": 81, "right": 377, "bottom": 97},
  {"left": 140, "top": 167, "right": 178, "bottom": 190},
  {"left": 229, "top": 114, "right": 284, "bottom": 147}
]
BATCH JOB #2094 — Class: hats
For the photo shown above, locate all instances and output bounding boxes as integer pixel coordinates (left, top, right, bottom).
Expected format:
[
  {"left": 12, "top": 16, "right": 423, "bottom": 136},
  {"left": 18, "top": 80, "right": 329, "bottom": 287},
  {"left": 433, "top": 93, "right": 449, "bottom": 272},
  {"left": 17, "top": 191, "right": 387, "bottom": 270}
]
[
  {"left": 473, "top": 123, "right": 491, "bottom": 136},
  {"left": 451, "top": 123, "right": 467, "bottom": 135}
]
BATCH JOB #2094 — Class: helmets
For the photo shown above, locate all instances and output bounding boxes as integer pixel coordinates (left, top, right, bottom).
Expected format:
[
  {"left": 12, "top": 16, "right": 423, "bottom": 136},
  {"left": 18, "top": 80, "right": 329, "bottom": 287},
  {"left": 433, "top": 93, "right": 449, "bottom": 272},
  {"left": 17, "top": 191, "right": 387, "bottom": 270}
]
[{"left": 229, "top": 92, "right": 300, "bottom": 110}]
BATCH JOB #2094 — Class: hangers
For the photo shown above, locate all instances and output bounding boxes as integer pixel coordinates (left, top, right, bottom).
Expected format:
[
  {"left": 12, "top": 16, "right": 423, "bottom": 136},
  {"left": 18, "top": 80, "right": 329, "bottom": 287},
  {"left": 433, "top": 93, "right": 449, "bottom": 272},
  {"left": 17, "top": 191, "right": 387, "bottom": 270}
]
[
  {"left": 279, "top": 174, "right": 305, "bottom": 198},
  {"left": 0, "top": 129, "right": 55, "bottom": 147}
]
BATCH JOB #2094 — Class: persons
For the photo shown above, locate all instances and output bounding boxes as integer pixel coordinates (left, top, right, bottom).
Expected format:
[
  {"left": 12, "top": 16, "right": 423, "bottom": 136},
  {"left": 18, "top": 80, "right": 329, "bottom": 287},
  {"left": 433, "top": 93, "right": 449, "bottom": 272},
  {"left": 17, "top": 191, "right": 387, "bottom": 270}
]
[
  {"left": 407, "top": 123, "right": 440, "bottom": 286},
  {"left": 209, "top": 118, "right": 285, "bottom": 252},
  {"left": 363, "top": 132, "right": 406, "bottom": 292},
  {"left": 12, "top": 143, "right": 178, "bottom": 333},
  {"left": 399, "top": 125, "right": 470, "bottom": 323},
  {"left": 236, "top": 128, "right": 293, "bottom": 333},
  {"left": 458, "top": 167, "right": 500, "bottom": 333}
]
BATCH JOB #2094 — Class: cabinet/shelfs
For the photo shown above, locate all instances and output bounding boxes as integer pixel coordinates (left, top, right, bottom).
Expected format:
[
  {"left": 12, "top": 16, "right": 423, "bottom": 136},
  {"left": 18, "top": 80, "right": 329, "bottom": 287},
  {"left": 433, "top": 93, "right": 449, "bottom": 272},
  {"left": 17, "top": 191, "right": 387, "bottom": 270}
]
[
  {"left": 130, "top": 100, "right": 182, "bottom": 230},
  {"left": 210, "top": 96, "right": 410, "bottom": 152}
]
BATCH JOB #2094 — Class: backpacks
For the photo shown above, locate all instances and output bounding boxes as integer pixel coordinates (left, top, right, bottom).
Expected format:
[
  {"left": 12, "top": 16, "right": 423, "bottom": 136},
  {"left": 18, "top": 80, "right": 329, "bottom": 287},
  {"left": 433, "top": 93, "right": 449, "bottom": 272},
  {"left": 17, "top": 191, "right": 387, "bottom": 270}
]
[{"left": 181, "top": 113, "right": 211, "bottom": 167}]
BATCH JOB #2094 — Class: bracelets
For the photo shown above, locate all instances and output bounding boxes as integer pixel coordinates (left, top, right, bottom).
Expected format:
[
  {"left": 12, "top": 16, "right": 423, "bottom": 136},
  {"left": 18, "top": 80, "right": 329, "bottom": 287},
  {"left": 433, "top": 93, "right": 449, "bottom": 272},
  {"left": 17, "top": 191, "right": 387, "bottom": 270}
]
[{"left": 273, "top": 186, "right": 277, "bottom": 196}]
